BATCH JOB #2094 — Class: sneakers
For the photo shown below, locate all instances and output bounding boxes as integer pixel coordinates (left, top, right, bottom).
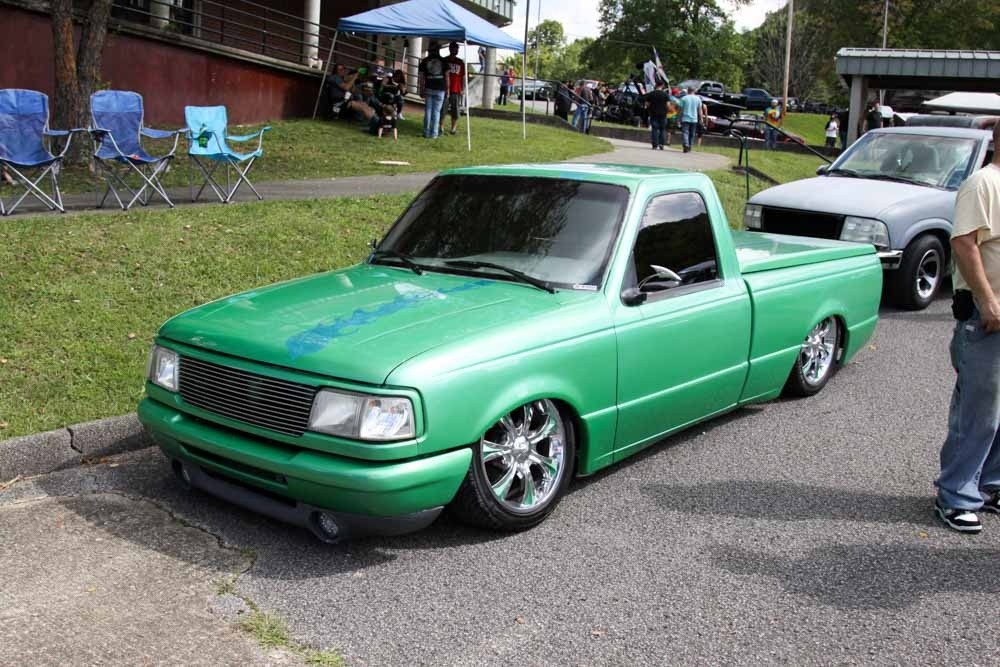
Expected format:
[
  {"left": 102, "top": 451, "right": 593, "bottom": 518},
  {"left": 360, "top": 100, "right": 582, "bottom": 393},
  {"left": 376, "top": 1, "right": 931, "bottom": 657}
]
[
  {"left": 933, "top": 497, "right": 983, "bottom": 531},
  {"left": 983, "top": 494, "right": 1000, "bottom": 514}
]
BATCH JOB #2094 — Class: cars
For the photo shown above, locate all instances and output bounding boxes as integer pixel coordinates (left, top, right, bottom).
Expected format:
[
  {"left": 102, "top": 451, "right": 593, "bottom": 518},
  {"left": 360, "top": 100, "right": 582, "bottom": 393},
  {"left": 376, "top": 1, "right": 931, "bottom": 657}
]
[
  {"left": 517, "top": 79, "right": 849, "bottom": 144},
  {"left": 742, "top": 115, "right": 1000, "bottom": 310}
]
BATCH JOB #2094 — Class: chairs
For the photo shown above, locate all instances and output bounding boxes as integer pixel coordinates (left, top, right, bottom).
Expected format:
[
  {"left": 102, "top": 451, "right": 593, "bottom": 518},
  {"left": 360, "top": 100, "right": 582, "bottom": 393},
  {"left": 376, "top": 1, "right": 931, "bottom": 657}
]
[
  {"left": 880, "top": 144, "right": 940, "bottom": 178},
  {"left": 185, "top": 105, "right": 272, "bottom": 204},
  {"left": 88, "top": 89, "right": 190, "bottom": 211},
  {"left": 0, "top": 88, "right": 87, "bottom": 216}
]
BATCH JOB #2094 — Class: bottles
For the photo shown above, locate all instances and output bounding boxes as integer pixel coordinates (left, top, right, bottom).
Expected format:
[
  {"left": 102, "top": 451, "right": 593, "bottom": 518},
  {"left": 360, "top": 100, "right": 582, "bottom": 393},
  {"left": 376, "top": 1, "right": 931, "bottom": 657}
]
[{"left": 198, "top": 123, "right": 207, "bottom": 147}]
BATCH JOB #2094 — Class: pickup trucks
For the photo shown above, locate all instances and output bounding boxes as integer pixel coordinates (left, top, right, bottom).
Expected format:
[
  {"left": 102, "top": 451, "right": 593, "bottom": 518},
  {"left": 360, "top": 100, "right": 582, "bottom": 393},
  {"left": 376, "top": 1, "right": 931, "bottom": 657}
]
[{"left": 136, "top": 162, "right": 883, "bottom": 539}]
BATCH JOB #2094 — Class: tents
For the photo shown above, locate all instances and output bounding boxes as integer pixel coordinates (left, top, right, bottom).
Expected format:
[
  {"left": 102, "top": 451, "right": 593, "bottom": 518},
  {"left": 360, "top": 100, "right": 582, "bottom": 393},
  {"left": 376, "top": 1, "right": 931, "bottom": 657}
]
[{"left": 312, "top": 0, "right": 526, "bottom": 151}]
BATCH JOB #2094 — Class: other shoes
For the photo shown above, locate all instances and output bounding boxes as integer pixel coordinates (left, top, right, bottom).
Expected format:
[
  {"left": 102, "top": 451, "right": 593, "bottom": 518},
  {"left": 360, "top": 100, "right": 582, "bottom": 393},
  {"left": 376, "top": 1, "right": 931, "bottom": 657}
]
[
  {"left": 659, "top": 144, "right": 663, "bottom": 150},
  {"left": 399, "top": 113, "right": 405, "bottom": 120}
]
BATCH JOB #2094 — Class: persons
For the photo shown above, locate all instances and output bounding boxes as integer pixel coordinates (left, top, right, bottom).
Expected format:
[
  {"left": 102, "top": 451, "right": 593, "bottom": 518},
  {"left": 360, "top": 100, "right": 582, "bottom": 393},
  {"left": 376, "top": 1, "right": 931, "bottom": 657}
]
[
  {"left": 764, "top": 98, "right": 785, "bottom": 150},
  {"left": 643, "top": 79, "right": 707, "bottom": 150},
  {"left": 824, "top": 99, "right": 896, "bottom": 160},
  {"left": 933, "top": 106, "right": 1000, "bottom": 533},
  {"left": 321, "top": 64, "right": 406, "bottom": 142},
  {"left": 418, "top": 39, "right": 449, "bottom": 140},
  {"left": 439, "top": 42, "right": 465, "bottom": 135},
  {"left": 479, "top": 45, "right": 487, "bottom": 73},
  {"left": 498, "top": 66, "right": 514, "bottom": 104},
  {"left": 555, "top": 78, "right": 609, "bottom": 135}
]
